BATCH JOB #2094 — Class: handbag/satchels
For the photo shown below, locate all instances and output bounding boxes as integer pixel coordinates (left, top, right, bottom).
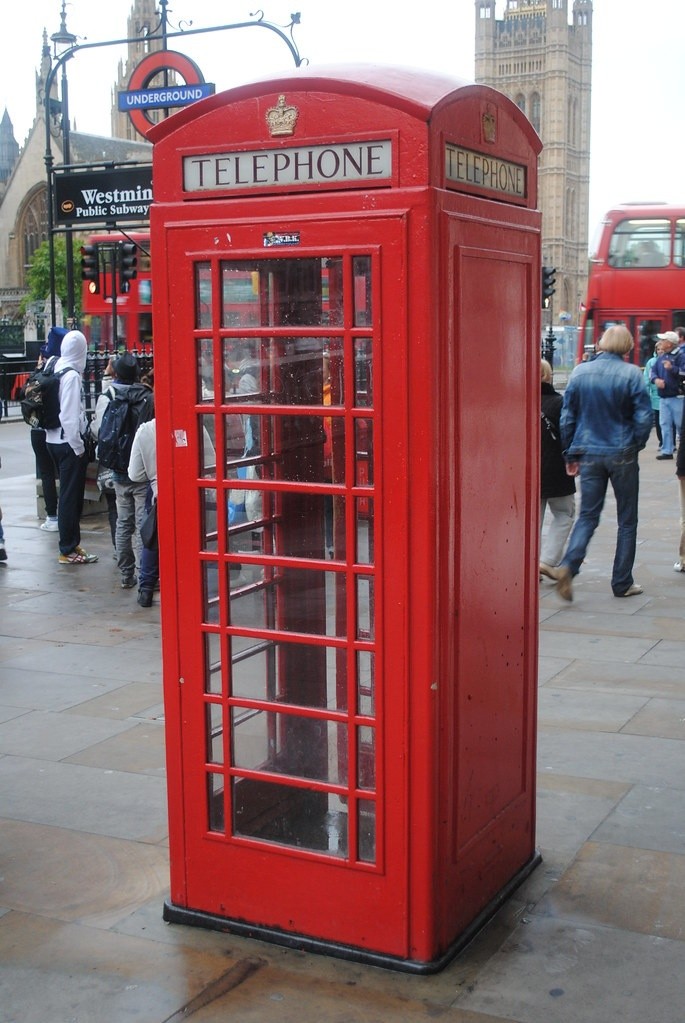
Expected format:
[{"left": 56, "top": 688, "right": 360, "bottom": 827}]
[
  {"left": 97, "top": 467, "right": 116, "bottom": 493},
  {"left": 82, "top": 429, "right": 97, "bottom": 463}
]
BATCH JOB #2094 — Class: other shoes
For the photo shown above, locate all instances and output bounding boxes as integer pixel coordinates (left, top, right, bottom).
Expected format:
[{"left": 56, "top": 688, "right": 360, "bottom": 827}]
[
  {"left": 137, "top": 591, "right": 153, "bottom": 607},
  {"left": 657, "top": 442, "right": 663, "bottom": 451},
  {"left": 673, "top": 562, "right": 685, "bottom": 571},
  {"left": 623, "top": 584, "right": 642, "bottom": 596},
  {"left": 538, "top": 562, "right": 559, "bottom": 579},
  {"left": 554, "top": 564, "right": 573, "bottom": 601},
  {"left": 0, "top": 548, "right": 8, "bottom": 560},
  {"left": 656, "top": 453, "right": 673, "bottom": 460},
  {"left": 229, "top": 574, "right": 245, "bottom": 588},
  {"left": 121, "top": 575, "right": 137, "bottom": 588},
  {"left": 154, "top": 579, "right": 161, "bottom": 591}
]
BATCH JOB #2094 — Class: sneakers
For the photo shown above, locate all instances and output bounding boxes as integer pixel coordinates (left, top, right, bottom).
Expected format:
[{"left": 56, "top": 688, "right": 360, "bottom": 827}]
[
  {"left": 39, "top": 515, "right": 59, "bottom": 532},
  {"left": 59, "top": 544, "right": 99, "bottom": 564}
]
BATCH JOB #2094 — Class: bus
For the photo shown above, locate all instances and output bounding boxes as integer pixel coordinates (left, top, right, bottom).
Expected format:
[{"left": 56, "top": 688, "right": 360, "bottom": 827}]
[
  {"left": 574, "top": 206, "right": 685, "bottom": 376},
  {"left": 77, "top": 232, "right": 332, "bottom": 392}
]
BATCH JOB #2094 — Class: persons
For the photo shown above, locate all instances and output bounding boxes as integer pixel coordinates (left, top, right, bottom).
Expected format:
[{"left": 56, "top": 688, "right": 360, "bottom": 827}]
[
  {"left": 581, "top": 337, "right": 603, "bottom": 364},
  {"left": 538, "top": 360, "right": 578, "bottom": 582},
  {"left": 556, "top": 325, "right": 653, "bottom": 601},
  {"left": 641, "top": 326, "right": 685, "bottom": 572},
  {"left": 0, "top": 457, "right": 8, "bottom": 560},
  {"left": 21, "top": 328, "right": 367, "bottom": 607}
]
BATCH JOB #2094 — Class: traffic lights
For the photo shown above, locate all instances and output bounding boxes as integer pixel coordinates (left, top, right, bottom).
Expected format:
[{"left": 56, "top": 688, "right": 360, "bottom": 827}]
[
  {"left": 80, "top": 241, "right": 99, "bottom": 281},
  {"left": 540, "top": 266, "right": 557, "bottom": 308},
  {"left": 118, "top": 240, "right": 138, "bottom": 281}
]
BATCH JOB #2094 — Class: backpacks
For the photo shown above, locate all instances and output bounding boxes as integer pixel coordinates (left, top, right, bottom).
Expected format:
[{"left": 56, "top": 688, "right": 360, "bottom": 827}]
[
  {"left": 21, "top": 356, "right": 74, "bottom": 429},
  {"left": 98, "top": 393, "right": 153, "bottom": 470}
]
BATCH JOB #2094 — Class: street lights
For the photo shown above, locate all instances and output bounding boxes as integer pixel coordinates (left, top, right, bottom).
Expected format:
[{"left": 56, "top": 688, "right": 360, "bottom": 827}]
[{"left": 49, "top": 0, "right": 79, "bottom": 330}]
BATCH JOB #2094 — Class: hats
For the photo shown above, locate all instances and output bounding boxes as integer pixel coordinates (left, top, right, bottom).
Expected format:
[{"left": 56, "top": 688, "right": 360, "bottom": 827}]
[
  {"left": 657, "top": 330, "right": 679, "bottom": 344},
  {"left": 111, "top": 350, "right": 141, "bottom": 379}
]
